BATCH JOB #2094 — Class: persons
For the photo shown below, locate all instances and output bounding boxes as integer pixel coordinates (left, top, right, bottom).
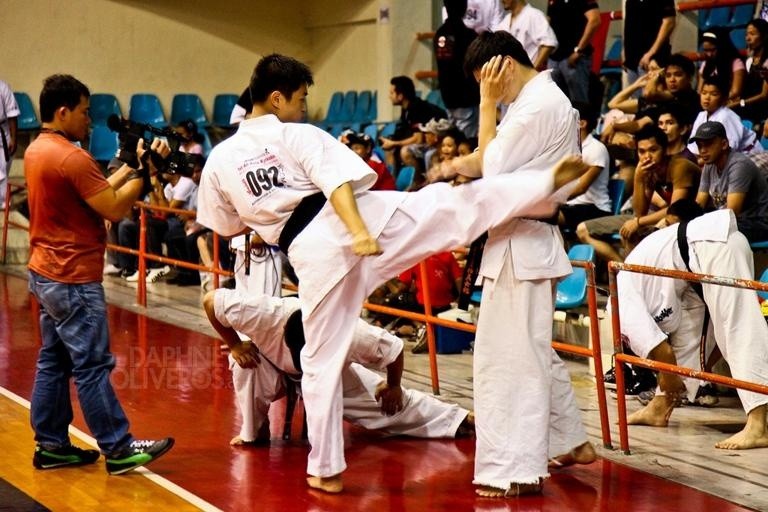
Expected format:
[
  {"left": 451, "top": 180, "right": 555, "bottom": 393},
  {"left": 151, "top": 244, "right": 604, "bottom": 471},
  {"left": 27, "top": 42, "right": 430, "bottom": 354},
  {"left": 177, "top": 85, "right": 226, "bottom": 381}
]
[
  {"left": 0, "top": 78, "right": 21, "bottom": 210},
  {"left": 104, "top": 0, "right": 768, "bottom": 497},
  {"left": 23, "top": 73, "right": 172, "bottom": 475}
]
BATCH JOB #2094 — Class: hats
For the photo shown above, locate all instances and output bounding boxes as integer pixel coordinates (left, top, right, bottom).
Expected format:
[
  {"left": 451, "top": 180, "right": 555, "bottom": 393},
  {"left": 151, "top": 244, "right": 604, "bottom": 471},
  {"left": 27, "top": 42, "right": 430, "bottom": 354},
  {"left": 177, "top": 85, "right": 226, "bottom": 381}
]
[
  {"left": 688, "top": 121, "right": 727, "bottom": 144},
  {"left": 346, "top": 132, "right": 376, "bottom": 151}
]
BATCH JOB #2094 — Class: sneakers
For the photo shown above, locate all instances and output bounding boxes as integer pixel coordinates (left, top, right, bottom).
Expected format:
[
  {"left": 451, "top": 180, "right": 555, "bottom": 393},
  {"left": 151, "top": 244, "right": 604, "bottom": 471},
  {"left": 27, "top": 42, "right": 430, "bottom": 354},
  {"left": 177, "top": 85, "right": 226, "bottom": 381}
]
[
  {"left": 592, "top": 361, "right": 633, "bottom": 390},
  {"left": 412, "top": 324, "right": 427, "bottom": 353},
  {"left": 105, "top": 437, "right": 174, "bottom": 475},
  {"left": 682, "top": 383, "right": 720, "bottom": 407},
  {"left": 636, "top": 383, "right": 680, "bottom": 406},
  {"left": 32, "top": 445, "right": 100, "bottom": 469},
  {"left": 103, "top": 263, "right": 171, "bottom": 283},
  {"left": 608, "top": 373, "right": 658, "bottom": 401}
]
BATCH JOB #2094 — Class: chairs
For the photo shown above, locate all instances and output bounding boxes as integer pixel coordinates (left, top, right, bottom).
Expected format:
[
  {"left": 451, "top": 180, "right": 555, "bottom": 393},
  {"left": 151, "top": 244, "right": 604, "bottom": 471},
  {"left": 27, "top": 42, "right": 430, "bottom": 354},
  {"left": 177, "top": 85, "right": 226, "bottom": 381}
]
[{"left": 11, "top": 0, "right": 766, "bottom": 311}]
